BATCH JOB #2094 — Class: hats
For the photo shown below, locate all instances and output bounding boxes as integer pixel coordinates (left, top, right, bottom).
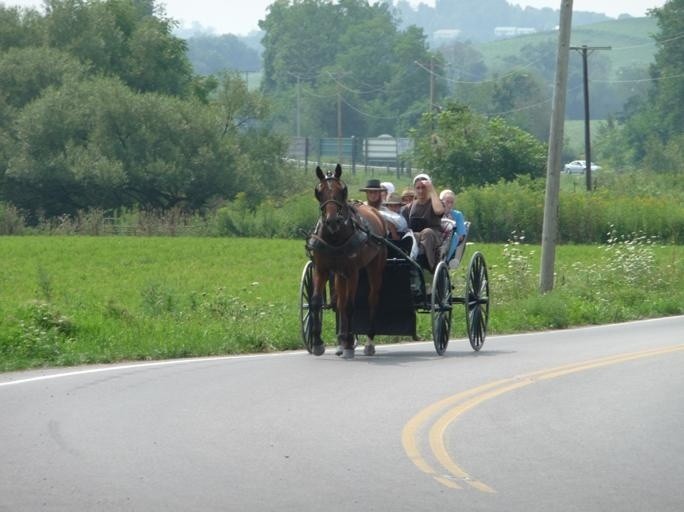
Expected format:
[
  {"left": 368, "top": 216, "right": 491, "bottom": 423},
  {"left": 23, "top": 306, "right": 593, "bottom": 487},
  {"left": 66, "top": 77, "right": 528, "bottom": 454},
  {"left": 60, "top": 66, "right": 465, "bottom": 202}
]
[
  {"left": 413, "top": 173, "right": 431, "bottom": 186},
  {"left": 380, "top": 182, "right": 395, "bottom": 202},
  {"left": 383, "top": 193, "right": 406, "bottom": 207},
  {"left": 359, "top": 179, "right": 386, "bottom": 192}
]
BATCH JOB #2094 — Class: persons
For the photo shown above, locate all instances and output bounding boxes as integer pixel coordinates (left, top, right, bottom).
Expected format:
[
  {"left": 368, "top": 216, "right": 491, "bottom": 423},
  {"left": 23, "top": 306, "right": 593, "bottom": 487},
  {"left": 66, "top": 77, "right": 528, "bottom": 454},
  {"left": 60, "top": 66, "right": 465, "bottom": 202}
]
[{"left": 358, "top": 174, "right": 467, "bottom": 269}]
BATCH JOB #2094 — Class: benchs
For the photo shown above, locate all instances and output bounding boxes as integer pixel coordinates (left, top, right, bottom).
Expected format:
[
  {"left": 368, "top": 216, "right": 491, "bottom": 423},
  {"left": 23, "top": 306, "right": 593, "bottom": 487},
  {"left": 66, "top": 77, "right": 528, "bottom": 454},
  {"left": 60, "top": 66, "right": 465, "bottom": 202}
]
[
  {"left": 362, "top": 201, "right": 454, "bottom": 259},
  {"left": 450, "top": 220, "right": 472, "bottom": 267}
]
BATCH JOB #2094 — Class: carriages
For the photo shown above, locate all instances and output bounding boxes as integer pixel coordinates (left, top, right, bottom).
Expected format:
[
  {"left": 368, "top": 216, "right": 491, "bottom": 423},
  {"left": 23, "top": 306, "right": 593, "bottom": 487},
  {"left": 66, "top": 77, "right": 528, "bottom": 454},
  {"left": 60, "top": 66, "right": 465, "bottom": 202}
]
[{"left": 297, "top": 162, "right": 491, "bottom": 356}]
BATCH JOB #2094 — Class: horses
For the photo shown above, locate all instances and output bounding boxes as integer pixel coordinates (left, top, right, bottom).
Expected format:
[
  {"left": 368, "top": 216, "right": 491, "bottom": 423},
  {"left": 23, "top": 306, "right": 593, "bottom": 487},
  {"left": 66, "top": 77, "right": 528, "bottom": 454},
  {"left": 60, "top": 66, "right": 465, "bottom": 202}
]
[{"left": 305, "top": 162, "right": 389, "bottom": 359}]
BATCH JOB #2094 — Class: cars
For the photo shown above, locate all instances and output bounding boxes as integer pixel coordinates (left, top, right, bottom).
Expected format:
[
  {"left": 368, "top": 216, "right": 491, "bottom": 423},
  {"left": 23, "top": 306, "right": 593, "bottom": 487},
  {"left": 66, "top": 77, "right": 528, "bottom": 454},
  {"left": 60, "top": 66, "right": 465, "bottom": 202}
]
[{"left": 563, "top": 160, "right": 602, "bottom": 175}]
